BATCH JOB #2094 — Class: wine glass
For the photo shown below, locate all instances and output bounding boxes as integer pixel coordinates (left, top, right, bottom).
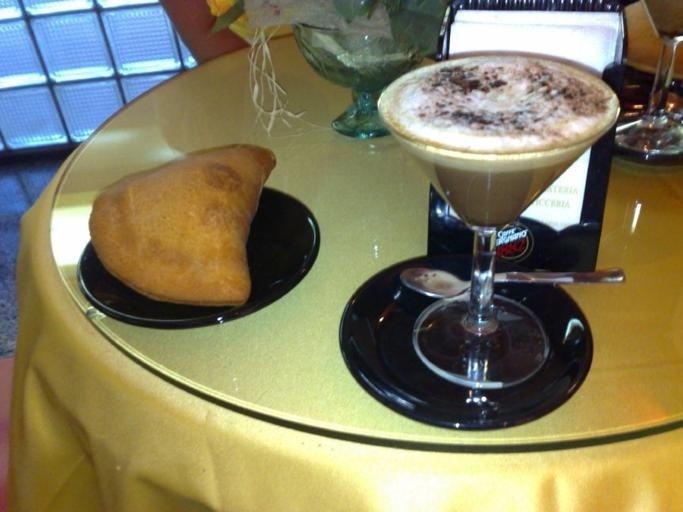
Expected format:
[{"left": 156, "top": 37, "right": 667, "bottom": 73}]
[
  {"left": 290, "top": 18, "right": 440, "bottom": 140},
  {"left": 374, "top": 47, "right": 625, "bottom": 390},
  {"left": 614, "top": 0, "right": 683, "bottom": 157}
]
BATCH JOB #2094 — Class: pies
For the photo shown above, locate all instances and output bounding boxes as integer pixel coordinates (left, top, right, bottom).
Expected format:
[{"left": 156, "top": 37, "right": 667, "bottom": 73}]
[{"left": 89, "top": 141, "right": 276, "bottom": 307}]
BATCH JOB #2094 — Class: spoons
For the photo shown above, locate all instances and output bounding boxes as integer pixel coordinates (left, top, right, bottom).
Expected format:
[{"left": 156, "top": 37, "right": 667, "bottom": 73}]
[{"left": 398, "top": 253, "right": 627, "bottom": 302}]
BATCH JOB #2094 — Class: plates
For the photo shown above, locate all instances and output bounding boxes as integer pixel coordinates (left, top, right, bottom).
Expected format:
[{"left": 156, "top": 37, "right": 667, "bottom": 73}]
[
  {"left": 337, "top": 255, "right": 598, "bottom": 435},
  {"left": 76, "top": 185, "right": 322, "bottom": 332}
]
